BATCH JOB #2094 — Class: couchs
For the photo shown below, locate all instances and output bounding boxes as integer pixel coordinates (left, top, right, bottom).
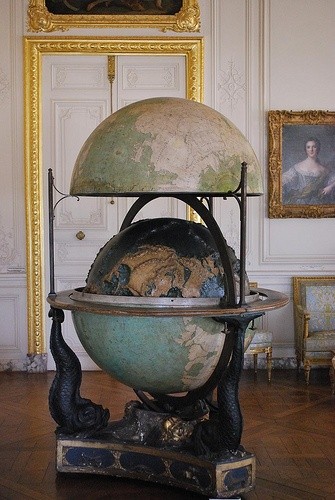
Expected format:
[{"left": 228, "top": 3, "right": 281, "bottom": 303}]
[{"left": 292, "top": 276, "right": 335, "bottom": 387}]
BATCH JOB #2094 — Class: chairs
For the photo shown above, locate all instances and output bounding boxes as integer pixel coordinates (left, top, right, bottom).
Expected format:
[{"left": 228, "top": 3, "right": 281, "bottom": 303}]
[{"left": 237, "top": 282, "right": 273, "bottom": 383}]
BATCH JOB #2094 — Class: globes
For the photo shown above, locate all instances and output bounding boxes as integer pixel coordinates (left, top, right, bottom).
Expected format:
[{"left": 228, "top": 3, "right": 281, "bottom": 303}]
[{"left": 43, "top": 96, "right": 292, "bottom": 498}]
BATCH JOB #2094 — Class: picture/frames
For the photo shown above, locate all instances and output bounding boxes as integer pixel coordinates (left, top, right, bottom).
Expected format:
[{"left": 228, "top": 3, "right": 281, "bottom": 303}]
[
  {"left": 267, "top": 109, "right": 335, "bottom": 219},
  {"left": 25, "top": 0, "right": 202, "bottom": 33}
]
